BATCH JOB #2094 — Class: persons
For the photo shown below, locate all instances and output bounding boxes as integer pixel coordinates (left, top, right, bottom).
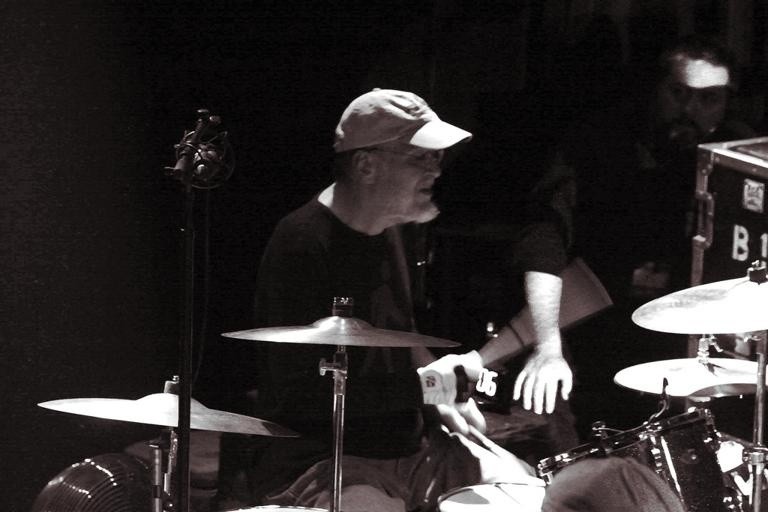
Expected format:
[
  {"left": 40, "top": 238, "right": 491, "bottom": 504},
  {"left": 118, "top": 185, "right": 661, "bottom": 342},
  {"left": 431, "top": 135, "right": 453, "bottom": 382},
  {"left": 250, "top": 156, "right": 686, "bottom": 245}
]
[
  {"left": 511, "top": 35, "right": 761, "bottom": 416},
  {"left": 252, "top": 87, "right": 499, "bottom": 431}
]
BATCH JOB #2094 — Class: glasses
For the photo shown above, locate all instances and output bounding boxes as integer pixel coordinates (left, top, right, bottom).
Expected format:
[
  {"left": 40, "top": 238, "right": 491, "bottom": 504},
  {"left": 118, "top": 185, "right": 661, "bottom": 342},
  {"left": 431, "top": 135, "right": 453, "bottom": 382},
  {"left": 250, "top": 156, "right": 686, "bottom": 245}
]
[{"left": 364, "top": 149, "right": 444, "bottom": 164}]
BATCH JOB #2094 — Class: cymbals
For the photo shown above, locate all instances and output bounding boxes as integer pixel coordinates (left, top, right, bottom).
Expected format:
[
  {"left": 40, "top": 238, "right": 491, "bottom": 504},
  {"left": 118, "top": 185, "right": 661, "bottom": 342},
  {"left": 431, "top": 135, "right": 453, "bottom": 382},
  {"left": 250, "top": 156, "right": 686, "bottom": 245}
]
[
  {"left": 631, "top": 274, "right": 768, "bottom": 335},
  {"left": 615, "top": 356, "right": 768, "bottom": 396},
  {"left": 38, "top": 392, "right": 301, "bottom": 439},
  {"left": 219, "top": 317, "right": 461, "bottom": 348}
]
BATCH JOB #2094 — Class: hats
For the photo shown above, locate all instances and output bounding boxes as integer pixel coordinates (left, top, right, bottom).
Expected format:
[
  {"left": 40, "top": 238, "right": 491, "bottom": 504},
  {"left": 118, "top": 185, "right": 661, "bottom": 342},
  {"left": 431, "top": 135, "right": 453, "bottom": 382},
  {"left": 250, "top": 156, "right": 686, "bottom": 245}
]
[
  {"left": 542, "top": 457, "right": 684, "bottom": 512},
  {"left": 668, "top": 35, "right": 741, "bottom": 95},
  {"left": 332, "top": 88, "right": 473, "bottom": 154}
]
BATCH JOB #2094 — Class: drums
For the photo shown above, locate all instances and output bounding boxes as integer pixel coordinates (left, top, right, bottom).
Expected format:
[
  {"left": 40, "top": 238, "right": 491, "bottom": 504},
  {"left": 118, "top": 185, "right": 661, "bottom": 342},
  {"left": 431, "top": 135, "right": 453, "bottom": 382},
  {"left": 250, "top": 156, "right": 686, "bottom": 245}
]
[{"left": 538, "top": 407, "right": 725, "bottom": 512}]
